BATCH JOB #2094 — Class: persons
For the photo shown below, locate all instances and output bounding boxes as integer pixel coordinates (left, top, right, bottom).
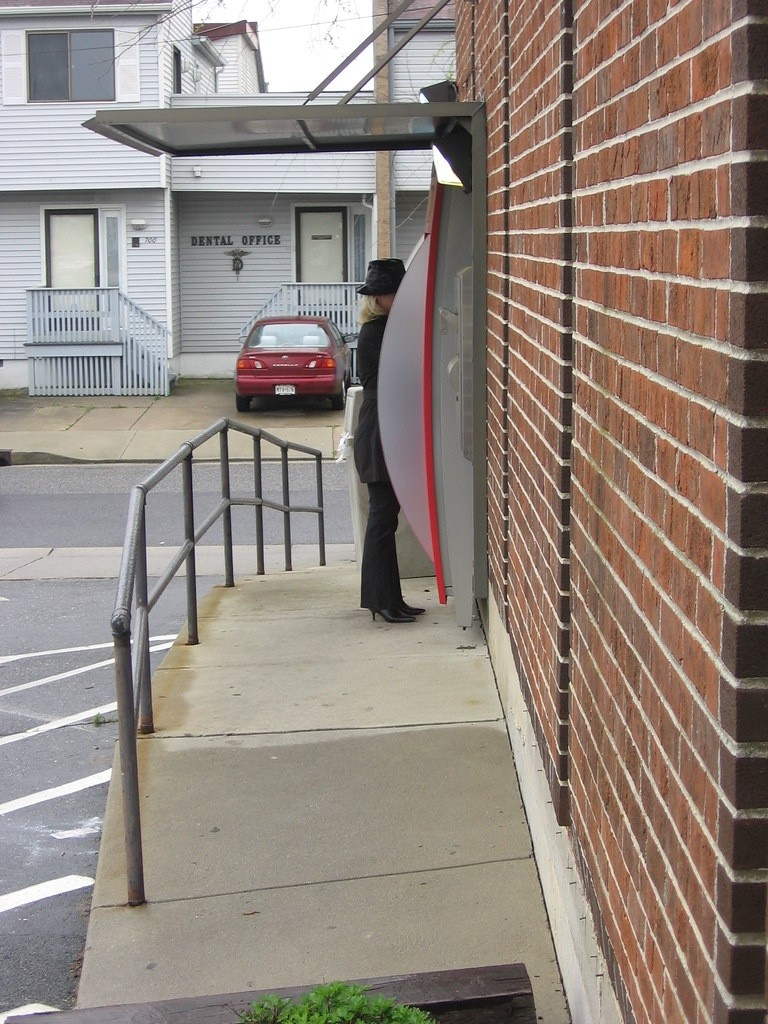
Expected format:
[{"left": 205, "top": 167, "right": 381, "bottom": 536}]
[{"left": 354, "top": 258, "right": 426, "bottom": 623}]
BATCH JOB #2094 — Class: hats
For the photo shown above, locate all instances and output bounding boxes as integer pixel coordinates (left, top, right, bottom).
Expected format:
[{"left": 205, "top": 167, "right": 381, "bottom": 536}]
[{"left": 355, "top": 258, "right": 407, "bottom": 295}]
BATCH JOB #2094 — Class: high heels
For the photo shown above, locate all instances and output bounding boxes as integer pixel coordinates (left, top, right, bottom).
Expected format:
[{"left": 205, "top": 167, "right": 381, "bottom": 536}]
[
  {"left": 369, "top": 606, "right": 416, "bottom": 623},
  {"left": 397, "top": 600, "right": 426, "bottom": 615}
]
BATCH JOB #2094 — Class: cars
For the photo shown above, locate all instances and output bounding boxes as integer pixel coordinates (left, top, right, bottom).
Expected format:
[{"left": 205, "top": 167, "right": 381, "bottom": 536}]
[{"left": 232, "top": 314, "right": 356, "bottom": 411}]
